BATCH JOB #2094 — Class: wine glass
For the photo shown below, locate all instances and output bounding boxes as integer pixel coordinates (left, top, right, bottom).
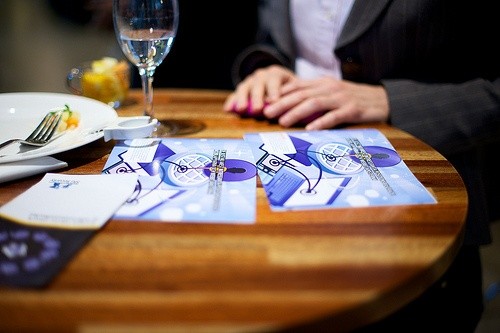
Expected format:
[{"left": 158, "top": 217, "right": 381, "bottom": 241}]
[{"left": 112, "top": 0, "right": 179, "bottom": 139}]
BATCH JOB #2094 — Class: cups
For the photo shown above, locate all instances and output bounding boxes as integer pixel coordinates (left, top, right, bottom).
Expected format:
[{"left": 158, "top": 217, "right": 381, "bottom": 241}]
[{"left": 65, "top": 58, "right": 130, "bottom": 110}]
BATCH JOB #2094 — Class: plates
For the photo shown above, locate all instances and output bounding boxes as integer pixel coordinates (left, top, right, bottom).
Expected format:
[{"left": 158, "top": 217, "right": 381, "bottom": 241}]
[{"left": 0, "top": 90, "right": 119, "bottom": 164}]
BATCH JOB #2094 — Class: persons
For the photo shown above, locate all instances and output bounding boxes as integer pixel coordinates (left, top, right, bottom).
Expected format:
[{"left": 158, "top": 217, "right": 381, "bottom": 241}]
[{"left": 223, "top": 0, "right": 500, "bottom": 333}]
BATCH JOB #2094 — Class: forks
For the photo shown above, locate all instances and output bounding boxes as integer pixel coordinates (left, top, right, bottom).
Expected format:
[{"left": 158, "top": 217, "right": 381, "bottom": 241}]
[{"left": 0, "top": 112, "right": 62, "bottom": 150}]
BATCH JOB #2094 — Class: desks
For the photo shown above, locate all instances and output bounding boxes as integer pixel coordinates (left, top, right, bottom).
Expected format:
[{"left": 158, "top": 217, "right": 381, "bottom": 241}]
[{"left": 0, "top": 90, "right": 468, "bottom": 333}]
